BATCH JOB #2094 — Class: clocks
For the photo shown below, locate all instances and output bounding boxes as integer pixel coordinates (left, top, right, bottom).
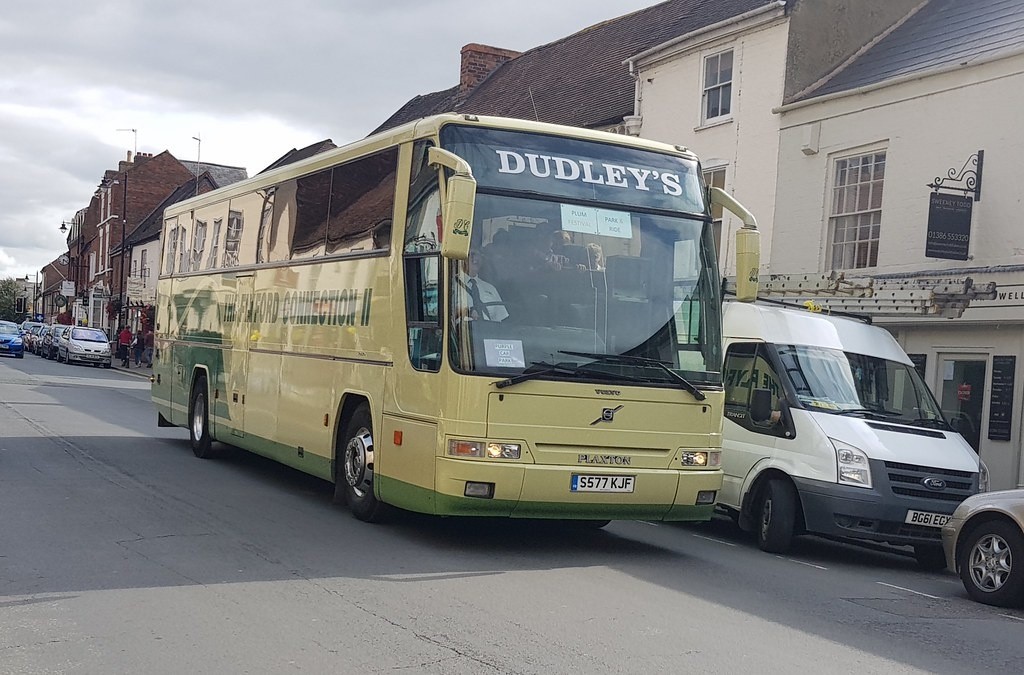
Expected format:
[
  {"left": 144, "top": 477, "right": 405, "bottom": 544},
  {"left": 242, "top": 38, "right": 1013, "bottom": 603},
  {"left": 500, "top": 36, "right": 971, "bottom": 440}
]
[{"left": 58, "top": 254, "right": 69, "bottom": 266}]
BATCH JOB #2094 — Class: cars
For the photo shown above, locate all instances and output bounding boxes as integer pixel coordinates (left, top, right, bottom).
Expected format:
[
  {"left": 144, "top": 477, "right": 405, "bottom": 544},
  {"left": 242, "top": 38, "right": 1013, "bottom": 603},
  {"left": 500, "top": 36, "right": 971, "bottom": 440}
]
[
  {"left": 18, "top": 321, "right": 68, "bottom": 360},
  {"left": 0, "top": 319, "right": 26, "bottom": 359},
  {"left": 56, "top": 325, "right": 117, "bottom": 368},
  {"left": 940, "top": 488, "right": 1024, "bottom": 611}
]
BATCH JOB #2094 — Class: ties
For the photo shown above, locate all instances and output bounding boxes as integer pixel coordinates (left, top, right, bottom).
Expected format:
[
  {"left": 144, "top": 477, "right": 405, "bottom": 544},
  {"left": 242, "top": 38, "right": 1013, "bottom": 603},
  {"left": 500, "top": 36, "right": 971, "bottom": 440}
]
[{"left": 470, "top": 278, "right": 483, "bottom": 320}]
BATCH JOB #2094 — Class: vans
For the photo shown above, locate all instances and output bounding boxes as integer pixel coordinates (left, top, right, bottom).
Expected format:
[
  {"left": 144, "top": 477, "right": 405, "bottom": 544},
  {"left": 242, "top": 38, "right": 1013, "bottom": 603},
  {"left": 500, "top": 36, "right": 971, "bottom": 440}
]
[{"left": 671, "top": 298, "right": 991, "bottom": 572}]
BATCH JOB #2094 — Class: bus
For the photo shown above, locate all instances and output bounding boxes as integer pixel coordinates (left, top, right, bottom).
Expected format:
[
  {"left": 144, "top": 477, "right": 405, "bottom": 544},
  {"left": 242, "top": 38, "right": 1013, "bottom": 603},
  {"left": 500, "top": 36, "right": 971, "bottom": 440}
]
[{"left": 148, "top": 112, "right": 762, "bottom": 530}]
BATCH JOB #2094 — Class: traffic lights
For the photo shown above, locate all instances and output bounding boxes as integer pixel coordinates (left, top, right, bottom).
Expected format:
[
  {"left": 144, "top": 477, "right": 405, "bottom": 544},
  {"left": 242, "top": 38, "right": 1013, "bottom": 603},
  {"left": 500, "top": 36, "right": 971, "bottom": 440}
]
[{"left": 15, "top": 297, "right": 25, "bottom": 314}]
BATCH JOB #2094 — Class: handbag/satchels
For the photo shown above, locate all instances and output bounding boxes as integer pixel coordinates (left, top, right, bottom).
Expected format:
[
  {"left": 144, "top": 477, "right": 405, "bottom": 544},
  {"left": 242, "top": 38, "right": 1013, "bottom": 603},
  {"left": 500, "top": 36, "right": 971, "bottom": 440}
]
[{"left": 131, "top": 335, "right": 137, "bottom": 346}]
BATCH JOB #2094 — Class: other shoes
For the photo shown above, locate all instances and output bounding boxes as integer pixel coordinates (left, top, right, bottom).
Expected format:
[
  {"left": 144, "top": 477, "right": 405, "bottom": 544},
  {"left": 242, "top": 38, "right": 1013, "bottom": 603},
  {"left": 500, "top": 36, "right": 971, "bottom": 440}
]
[
  {"left": 147, "top": 363, "right": 153, "bottom": 368},
  {"left": 138, "top": 362, "right": 142, "bottom": 367}
]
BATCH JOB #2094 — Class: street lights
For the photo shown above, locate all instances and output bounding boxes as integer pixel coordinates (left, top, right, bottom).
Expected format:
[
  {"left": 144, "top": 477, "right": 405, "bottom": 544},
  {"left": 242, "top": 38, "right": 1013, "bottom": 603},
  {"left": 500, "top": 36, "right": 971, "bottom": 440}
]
[
  {"left": 192, "top": 131, "right": 202, "bottom": 195},
  {"left": 23, "top": 283, "right": 36, "bottom": 321},
  {"left": 58, "top": 215, "right": 81, "bottom": 324},
  {"left": 96, "top": 171, "right": 128, "bottom": 359},
  {"left": 24, "top": 270, "right": 39, "bottom": 322}
]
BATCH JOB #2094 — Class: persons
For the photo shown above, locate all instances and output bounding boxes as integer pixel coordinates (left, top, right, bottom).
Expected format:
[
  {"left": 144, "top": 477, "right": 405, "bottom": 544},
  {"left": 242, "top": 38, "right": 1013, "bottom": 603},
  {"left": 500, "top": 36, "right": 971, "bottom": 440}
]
[
  {"left": 118, "top": 325, "right": 154, "bottom": 369},
  {"left": 451, "top": 223, "right": 605, "bottom": 329}
]
